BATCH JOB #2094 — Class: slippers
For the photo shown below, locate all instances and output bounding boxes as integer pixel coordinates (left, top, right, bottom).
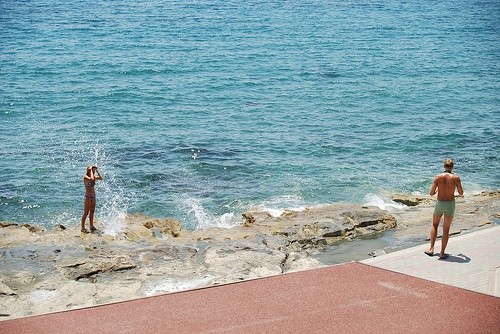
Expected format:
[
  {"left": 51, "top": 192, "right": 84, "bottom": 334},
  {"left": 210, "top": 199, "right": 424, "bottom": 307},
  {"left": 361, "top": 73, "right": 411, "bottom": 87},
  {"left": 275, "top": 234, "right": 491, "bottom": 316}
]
[
  {"left": 425, "top": 251, "right": 434, "bottom": 255},
  {"left": 440, "top": 253, "right": 449, "bottom": 259}
]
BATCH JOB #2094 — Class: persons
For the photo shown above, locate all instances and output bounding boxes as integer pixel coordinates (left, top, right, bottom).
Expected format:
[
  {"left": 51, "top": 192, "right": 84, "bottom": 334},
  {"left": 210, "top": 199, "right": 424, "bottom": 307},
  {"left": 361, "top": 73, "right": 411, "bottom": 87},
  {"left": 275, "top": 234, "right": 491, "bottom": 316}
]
[
  {"left": 80, "top": 166, "right": 103, "bottom": 233},
  {"left": 425, "top": 159, "right": 464, "bottom": 259}
]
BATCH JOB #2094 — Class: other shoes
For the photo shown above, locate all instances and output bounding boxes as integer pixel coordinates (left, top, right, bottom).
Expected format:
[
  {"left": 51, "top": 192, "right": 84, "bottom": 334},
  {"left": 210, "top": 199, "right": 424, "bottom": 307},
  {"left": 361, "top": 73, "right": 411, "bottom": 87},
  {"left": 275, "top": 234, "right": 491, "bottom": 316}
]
[
  {"left": 90, "top": 226, "right": 97, "bottom": 230},
  {"left": 81, "top": 228, "right": 89, "bottom": 234}
]
